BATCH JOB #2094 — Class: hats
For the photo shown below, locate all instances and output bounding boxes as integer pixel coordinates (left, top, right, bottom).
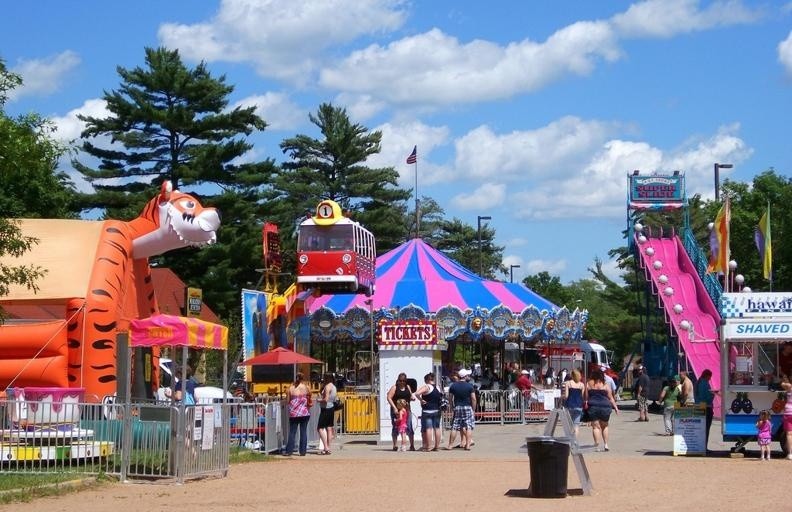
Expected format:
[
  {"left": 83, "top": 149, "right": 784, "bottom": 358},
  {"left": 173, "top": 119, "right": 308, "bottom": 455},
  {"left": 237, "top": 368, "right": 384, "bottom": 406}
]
[
  {"left": 672, "top": 375, "right": 681, "bottom": 381},
  {"left": 521, "top": 370, "right": 530, "bottom": 374},
  {"left": 458, "top": 369, "right": 472, "bottom": 378}
]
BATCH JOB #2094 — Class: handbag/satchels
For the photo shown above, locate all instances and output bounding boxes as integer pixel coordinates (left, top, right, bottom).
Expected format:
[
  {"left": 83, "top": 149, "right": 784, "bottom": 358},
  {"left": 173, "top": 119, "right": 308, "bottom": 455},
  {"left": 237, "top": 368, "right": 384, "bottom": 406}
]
[
  {"left": 677, "top": 395, "right": 685, "bottom": 404},
  {"left": 333, "top": 400, "right": 343, "bottom": 410},
  {"left": 307, "top": 391, "right": 313, "bottom": 406},
  {"left": 584, "top": 400, "right": 589, "bottom": 410},
  {"left": 440, "top": 393, "right": 448, "bottom": 411},
  {"left": 183, "top": 392, "right": 196, "bottom": 406}
]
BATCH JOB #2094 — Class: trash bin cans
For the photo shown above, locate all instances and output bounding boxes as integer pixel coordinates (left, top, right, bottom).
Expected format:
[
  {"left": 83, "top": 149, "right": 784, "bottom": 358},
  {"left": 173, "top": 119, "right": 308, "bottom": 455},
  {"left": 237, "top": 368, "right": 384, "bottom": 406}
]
[{"left": 526, "top": 436, "right": 572, "bottom": 497}]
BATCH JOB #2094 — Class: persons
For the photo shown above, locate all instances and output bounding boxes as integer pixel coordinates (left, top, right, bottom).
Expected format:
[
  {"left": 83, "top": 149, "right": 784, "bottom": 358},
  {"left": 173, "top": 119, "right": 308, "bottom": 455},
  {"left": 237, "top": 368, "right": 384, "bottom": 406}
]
[
  {"left": 659, "top": 369, "right": 695, "bottom": 436},
  {"left": 561, "top": 362, "right": 625, "bottom": 451},
  {"left": 755, "top": 392, "right": 792, "bottom": 460},
  {"left": 442, "top": 362, "right": 571, "bottom": 419},
  {"left": 386, "top": 368, "right": 478, "bottom": 451},
  {"left": 282, "top": 372, "right": 314, "bottom": 456},
  {"left": 164, "top": 363, "right": 198, "bottom": 467},
  {"left": 316, "top": 372, "right": 338, "bottom": 455},
  {"left": 631, "top": 362, "right": 651, "bottom": 423},
  {"left": 693, "top": 369, "right": 715, "bottom": 454},
  {"left": 732, "top": 366, "right": 792, "bottom": 391}
]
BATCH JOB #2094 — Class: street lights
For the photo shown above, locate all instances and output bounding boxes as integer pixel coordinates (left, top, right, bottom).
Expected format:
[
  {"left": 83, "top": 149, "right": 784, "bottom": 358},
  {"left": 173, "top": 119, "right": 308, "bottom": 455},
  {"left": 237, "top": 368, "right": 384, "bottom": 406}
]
[
  {"left": 478, "top": 215, "right": 492, "bottom": 278},
  {"left": 714, "top": 163, "right": 734, "bottom": 204},
  {"left": 510, "top": 264, "right": 520, "bottom": 283}
]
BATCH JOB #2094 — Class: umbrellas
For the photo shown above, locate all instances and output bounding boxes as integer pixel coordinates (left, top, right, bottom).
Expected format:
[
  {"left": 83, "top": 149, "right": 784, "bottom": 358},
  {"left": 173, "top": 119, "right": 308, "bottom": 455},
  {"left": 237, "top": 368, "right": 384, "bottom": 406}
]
[{"left": 239, "top": 346, "right": 324, "bottom": 399}]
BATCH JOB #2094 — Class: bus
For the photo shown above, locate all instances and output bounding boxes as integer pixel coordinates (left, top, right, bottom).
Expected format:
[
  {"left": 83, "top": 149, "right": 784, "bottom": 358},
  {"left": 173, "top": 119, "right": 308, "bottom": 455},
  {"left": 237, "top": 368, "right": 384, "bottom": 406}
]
[
  {"left": 504, "top": 342, "right": 615, "bottom": 384},
  {"left": 296, "top": 200, "right": 378, "bottom": 298}
]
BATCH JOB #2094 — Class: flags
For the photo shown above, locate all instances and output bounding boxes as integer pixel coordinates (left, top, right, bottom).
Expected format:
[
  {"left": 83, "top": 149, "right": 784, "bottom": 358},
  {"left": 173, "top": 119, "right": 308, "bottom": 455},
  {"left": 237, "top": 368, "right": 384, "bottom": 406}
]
[
  {"left": 406, "top": 146, "right": 418, "bottom": 163},
  {"left": 751, "top": 205, "right": 771, "bottom": 278},
  {"left": 707, "top": 203, "right": 731, "bottom": 278}
]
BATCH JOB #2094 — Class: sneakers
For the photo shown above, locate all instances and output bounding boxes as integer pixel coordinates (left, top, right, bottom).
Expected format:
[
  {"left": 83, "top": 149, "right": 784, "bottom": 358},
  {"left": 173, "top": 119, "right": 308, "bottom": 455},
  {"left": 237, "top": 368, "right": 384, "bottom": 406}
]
[
  {"left": 321, "top": 450, "right": 331, "bottom": 455},
  {"left": 393, "top": 440, "right": 474, "bottom": 452}
]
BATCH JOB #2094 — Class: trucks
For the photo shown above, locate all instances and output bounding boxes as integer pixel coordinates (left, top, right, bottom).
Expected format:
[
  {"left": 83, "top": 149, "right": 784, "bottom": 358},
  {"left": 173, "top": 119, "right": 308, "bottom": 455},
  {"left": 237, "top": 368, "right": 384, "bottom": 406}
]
[{"left": 157, "top": 356, "right": 234, "bottom": 423}]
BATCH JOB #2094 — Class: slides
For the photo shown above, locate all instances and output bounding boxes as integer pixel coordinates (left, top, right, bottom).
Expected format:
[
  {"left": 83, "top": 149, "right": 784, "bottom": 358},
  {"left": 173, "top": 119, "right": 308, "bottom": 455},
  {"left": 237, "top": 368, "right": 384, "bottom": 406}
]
[{"left": 635, "top": 230, "right": 737, "bottom": 420}]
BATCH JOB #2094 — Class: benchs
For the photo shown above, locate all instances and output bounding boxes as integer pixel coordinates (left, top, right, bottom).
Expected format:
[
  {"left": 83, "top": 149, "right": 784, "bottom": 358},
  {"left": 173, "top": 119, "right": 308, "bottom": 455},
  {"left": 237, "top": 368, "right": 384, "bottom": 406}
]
[{"left": 721, "top": 412, "right": 786, "bottom": 452}]
